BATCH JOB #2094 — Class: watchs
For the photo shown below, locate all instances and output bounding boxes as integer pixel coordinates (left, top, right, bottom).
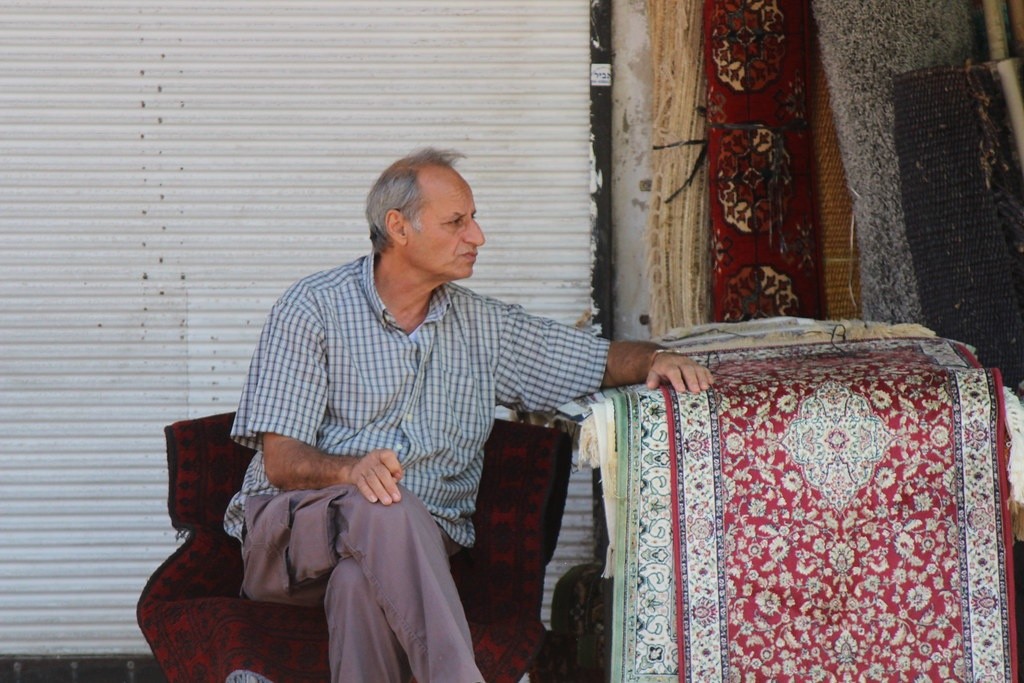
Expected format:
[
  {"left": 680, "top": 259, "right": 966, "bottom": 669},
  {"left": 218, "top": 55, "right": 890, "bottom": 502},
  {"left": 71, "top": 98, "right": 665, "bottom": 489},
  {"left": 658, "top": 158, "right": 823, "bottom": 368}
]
[{"left": 649, "top": 347, "right": 680, "bottom": 370}]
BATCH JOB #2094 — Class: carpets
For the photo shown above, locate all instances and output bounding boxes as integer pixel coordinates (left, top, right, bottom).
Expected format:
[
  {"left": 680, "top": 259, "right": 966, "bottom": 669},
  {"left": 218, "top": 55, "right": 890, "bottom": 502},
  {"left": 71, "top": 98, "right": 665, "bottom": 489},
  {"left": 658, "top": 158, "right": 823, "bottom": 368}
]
[{"left": 582, "top": 0, "right": 1024, "bottom": 683}]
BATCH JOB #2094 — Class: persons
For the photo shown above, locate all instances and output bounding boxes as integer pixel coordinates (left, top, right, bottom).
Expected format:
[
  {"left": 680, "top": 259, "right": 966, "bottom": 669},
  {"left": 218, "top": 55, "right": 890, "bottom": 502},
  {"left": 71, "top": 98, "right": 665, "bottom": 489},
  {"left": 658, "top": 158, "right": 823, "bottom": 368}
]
[{"left": 236, "top": 148, "right": 716, "bottom": 683}]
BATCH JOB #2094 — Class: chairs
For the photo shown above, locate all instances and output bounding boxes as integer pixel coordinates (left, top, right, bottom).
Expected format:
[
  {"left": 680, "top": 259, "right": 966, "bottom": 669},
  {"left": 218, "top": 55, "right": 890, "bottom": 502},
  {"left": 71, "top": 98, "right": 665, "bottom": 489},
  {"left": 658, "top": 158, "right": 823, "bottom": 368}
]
[{"left": 136, "top": 409, "right": 574, "bottom": 683}]
